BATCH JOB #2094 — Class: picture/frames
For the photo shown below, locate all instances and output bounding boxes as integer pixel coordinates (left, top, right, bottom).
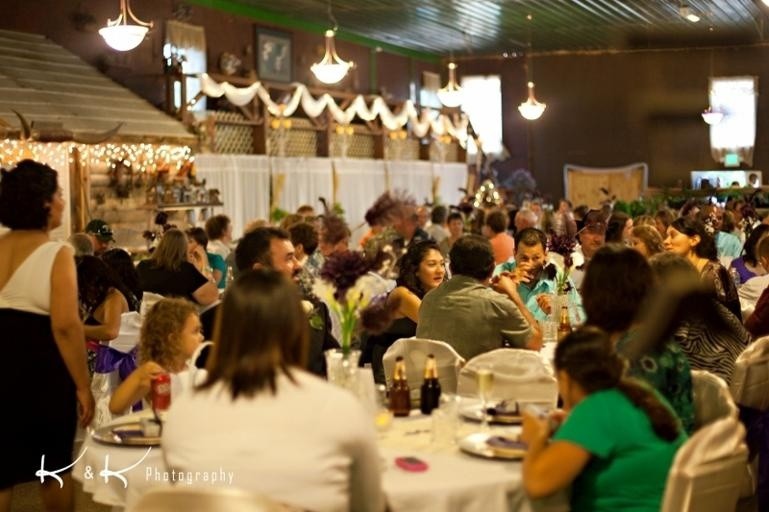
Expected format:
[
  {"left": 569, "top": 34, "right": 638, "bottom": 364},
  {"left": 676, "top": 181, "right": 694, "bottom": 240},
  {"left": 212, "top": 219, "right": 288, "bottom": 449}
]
[{"left": 253, "top": 24, "right": 294, "bottom": 83}]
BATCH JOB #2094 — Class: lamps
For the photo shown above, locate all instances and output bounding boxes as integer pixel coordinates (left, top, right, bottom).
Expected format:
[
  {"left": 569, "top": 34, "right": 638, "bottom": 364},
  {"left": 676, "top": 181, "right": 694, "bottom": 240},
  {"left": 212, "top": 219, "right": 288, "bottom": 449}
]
[
  {"left": 309, "top": 0, "right": 354, "bottom": 84},
  {"left": 517, "top": 13, "right": 547, "bottom": 121},
  {"left": 436, "top": 23, "right": 466, "bottom": 108},
  {"left": 97, "top": 0, "right": 154, "bottom": 51},
  {"left": 701, "top": 25, "right": 723, "bottom": 125}
]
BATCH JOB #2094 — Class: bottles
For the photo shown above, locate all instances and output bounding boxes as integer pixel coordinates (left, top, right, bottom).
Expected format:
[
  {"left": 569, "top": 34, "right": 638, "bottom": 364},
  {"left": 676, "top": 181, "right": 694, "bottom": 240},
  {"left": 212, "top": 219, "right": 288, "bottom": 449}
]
[
  {"left": 419, "top": 352, "right": 440, "bottom": 414},
  {"left": 225, "top": 266, "right": 235, "bottom": 290},
  {"left": 555, "top": 305, "right": 570, "bottom": 341},
  {"left": 150, "top": 371, "right": 171, "bottom": 410},
  {"left": 388, "top": 356, "right": 413, "bottom": 417}
]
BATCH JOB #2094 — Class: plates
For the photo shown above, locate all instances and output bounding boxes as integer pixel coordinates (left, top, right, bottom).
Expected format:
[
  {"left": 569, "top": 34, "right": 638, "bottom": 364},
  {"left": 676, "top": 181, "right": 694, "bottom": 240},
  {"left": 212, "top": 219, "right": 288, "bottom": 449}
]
[
  {"left": 458, "top": 400, "right": 550, "bottom": 425},
  {"left": 458, "top": 425, "right": 532, "bottom": 460},
  {"left": 90, "top": 418, "right": 164, "bottom": 447}
]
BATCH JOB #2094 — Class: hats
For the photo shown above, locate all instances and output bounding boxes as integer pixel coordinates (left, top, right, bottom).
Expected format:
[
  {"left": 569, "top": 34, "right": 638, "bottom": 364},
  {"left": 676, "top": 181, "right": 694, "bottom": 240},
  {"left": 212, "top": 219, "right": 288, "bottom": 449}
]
[{"left": 84, "top": 219, "right": 116, "bottom": 243}]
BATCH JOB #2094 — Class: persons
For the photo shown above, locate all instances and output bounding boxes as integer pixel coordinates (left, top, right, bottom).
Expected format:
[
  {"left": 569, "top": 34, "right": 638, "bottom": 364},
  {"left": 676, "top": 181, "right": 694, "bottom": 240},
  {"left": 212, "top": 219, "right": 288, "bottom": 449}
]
[
  {"left": 161, "top": 267, "right": 387, "bottom": 511},
  {"left": 376, "top": 172, "right": 769, "bottom": 437},
  {"left": 1, "top": 160, "right": 95, "bottom": 511},
  {"left": 516, "top": 331, "right": 686, "bottom": 511},
  {"left": 72, "top": 203, "right": 376, "bottom": 410}
]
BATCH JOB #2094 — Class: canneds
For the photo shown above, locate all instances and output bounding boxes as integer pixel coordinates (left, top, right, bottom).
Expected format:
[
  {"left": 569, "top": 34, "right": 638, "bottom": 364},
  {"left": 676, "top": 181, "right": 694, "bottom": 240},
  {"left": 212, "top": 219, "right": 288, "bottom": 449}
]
[{"left": 148, "top": 373, "right": 170, "bottom": 411}]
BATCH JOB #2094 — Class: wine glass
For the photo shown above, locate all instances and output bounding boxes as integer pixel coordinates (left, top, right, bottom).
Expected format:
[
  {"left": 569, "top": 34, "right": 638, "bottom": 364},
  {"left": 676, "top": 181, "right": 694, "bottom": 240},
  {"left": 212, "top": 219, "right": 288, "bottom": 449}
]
[{"left": 473, "top": 362, "right": 495, "bottom": 432}]
